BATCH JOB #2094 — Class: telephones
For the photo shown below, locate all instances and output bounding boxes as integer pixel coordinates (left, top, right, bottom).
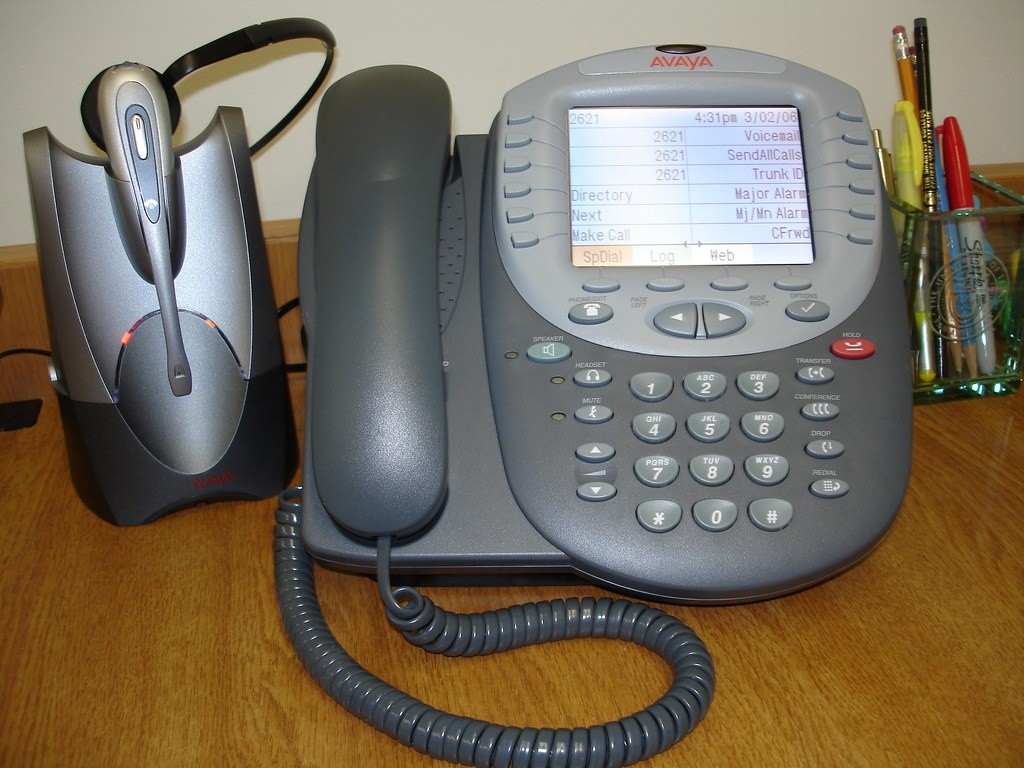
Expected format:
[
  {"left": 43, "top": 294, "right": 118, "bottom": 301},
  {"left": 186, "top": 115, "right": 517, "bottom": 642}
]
[{"left": 296, "top": 42, "right": 917, "bottom": 605}]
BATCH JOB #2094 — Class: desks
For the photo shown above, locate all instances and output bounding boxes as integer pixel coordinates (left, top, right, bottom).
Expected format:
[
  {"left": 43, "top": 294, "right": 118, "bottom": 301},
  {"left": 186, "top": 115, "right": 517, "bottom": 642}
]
[{"left": 0, "top": 158, "right": 1024, "bottom": 768}]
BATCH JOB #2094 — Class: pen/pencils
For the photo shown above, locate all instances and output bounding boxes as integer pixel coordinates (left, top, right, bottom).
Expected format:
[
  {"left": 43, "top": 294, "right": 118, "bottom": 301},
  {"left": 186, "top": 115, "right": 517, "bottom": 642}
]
[{"left": 871, "top": 18, "right": 996, "bottom": 384}]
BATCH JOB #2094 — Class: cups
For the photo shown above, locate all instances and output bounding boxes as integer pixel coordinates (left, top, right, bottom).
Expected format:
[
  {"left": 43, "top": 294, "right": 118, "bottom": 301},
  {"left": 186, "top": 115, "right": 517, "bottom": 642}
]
[{"left": 885, "top": 169, "right": 1024, "bottom": 401}]
